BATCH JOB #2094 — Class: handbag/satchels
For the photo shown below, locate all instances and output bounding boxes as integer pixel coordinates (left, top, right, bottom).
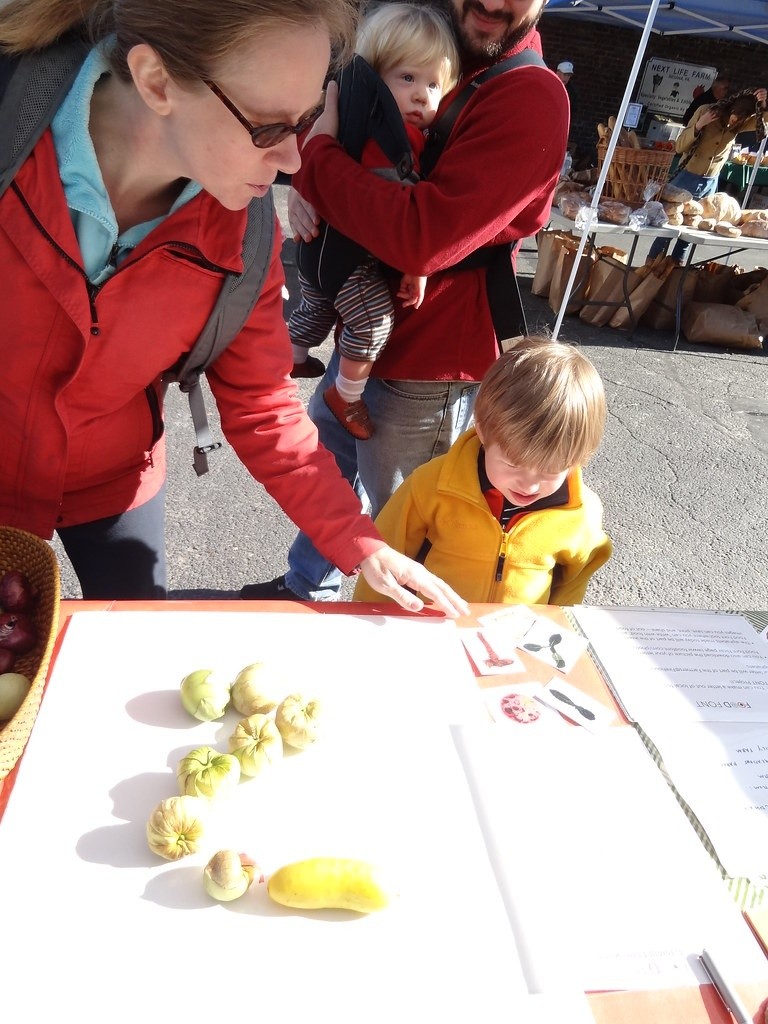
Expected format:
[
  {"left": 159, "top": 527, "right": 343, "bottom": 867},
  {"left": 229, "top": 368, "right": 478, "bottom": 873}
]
[{"left": 530, "top": 220, "right": 768, "bottom": 350}]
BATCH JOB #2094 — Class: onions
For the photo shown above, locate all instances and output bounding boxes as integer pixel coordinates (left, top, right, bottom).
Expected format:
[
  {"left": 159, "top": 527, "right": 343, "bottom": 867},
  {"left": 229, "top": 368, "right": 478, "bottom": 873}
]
[{"left": 0, "top": 567, "right": 37, "bottom": 719}]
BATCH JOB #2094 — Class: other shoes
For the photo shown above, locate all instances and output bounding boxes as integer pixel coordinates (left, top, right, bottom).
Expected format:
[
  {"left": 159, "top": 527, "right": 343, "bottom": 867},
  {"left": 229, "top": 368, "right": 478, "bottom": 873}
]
[
  {"left": 290, "top": 355, "right": 325, "bottom": 378},
  {"left": 323, "top": 382, "right": 373, "bottom": 440}
]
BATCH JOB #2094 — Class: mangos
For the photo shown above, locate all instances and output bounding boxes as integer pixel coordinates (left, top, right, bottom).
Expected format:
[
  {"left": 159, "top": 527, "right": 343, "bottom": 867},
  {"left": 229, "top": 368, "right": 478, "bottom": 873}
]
[{"left": 266, "top": 856, "right": 389, "bottom": 914}]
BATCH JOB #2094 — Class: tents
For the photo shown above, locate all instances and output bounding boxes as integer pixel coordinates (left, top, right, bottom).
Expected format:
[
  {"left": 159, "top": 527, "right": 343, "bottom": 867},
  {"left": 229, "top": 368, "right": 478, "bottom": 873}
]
[{"left": 536, "top": 0, "right": 768, "bottom": 346}]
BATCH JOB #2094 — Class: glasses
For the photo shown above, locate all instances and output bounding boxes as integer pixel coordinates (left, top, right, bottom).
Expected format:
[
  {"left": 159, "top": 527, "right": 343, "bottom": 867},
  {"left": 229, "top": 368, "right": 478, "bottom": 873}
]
[
  {"left": 563, "top": 73, "right": 573, "bottom": 76},
  {"left": 188, "top": 64, "right": 326, "bottom": 149}
]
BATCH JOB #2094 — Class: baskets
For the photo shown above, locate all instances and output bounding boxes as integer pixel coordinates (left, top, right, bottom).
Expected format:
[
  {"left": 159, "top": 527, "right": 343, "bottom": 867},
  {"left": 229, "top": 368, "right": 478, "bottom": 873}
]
[{"left": 0, "top": 525, "right": 61, "bottom": 780}]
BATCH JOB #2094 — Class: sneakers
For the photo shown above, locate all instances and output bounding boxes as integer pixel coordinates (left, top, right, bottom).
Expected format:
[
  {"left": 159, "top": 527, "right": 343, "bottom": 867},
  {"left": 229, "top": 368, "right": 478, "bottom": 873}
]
[{"left": 239, "top": 574, "right": 306, "bottom": 600}]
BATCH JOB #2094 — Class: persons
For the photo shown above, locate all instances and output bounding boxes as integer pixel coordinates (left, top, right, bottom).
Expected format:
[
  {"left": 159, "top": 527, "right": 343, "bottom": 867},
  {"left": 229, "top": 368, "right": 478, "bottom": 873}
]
[
  {"left": 647, "top": 76, "right": 768, "bottom": 263},
  {"left": 0, "top": 0, "right": 612, "bottom": 619}
]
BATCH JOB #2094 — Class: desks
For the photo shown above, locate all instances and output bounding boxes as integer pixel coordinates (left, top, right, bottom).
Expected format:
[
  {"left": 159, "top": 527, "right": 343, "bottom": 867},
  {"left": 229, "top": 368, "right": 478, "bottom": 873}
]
[
  {"left": 720, "top": 160, "right": 768, "bottom": 210},
  {"left": 0, "top": 598, "right": 768, "bottom": 1024},
  {"left": 518, "top": 206, "right": 768, "bottom": 351}
]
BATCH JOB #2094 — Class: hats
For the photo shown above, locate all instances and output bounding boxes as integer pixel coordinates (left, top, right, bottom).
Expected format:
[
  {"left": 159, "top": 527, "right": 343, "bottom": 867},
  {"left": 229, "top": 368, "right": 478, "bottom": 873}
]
[{"left": 557, "top": 62, "right": 574, "bottom": 73}]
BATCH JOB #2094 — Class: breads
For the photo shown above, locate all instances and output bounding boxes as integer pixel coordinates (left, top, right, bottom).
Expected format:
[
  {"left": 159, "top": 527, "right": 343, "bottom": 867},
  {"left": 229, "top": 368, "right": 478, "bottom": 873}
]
[{"left": 552, "top": 117, "right": 768, "bottom": 240}]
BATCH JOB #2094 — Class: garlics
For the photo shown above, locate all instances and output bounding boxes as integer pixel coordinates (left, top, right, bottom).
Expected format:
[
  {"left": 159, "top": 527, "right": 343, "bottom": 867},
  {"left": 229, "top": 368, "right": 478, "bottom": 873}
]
[{"left": 148, "top": 665, "right": 326, "bottom": 901}]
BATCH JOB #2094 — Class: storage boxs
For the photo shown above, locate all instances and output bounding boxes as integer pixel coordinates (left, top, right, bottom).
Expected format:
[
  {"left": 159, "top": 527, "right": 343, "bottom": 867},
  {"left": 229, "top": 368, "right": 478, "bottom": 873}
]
[
  {"left": 646, "top": 120, "right": 686, "bottom": 142},
  {"left": 749, "top": 194, "right": 768, "bottom": 210}
]
